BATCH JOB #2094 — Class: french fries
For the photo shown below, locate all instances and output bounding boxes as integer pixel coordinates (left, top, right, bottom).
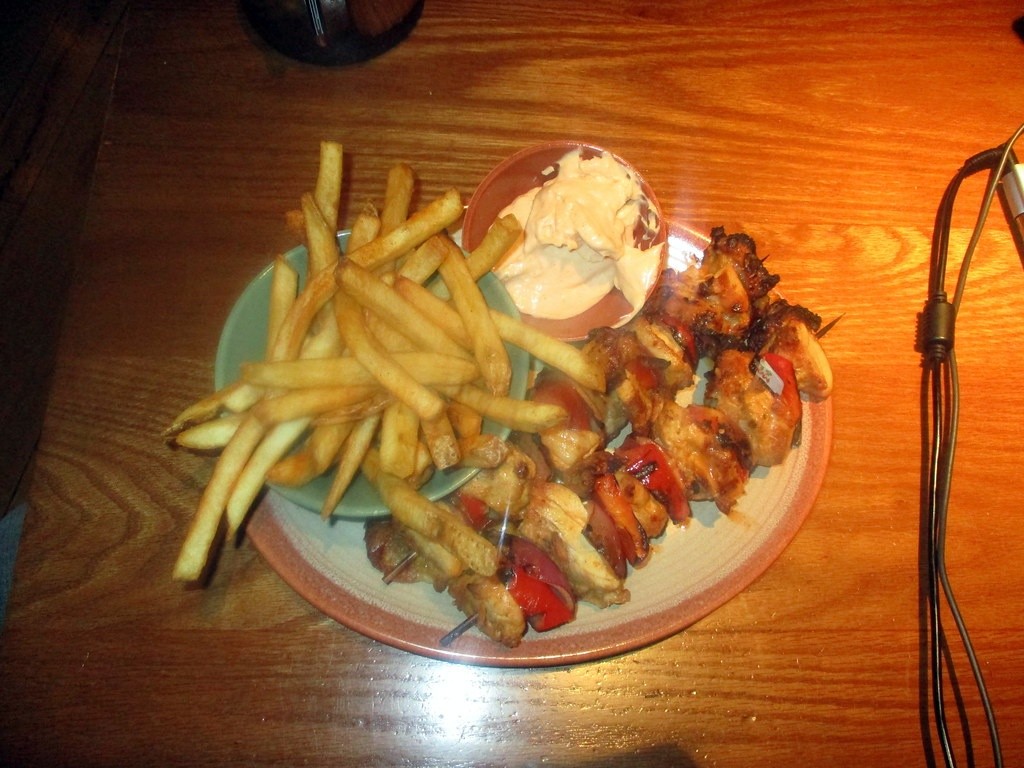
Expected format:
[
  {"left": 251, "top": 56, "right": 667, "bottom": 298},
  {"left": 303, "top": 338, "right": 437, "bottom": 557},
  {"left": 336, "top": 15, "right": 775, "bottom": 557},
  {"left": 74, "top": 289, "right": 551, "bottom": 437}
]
[{"left": 154, "top": 137, "right": 608, "bottom": 585}]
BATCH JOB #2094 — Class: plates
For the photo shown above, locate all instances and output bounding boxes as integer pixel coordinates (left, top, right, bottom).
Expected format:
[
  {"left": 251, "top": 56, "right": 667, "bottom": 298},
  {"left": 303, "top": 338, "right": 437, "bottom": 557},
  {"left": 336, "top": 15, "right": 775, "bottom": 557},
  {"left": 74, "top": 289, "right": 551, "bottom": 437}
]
[
  {"left": 245, "top": 216, "right": 832, "bottom": 669},
  {"left": 461, "top": 140, "right": 667, "bottom": 342},
  {"left": 213, "top": 229, "right": 529, "bottom": 520}
]
